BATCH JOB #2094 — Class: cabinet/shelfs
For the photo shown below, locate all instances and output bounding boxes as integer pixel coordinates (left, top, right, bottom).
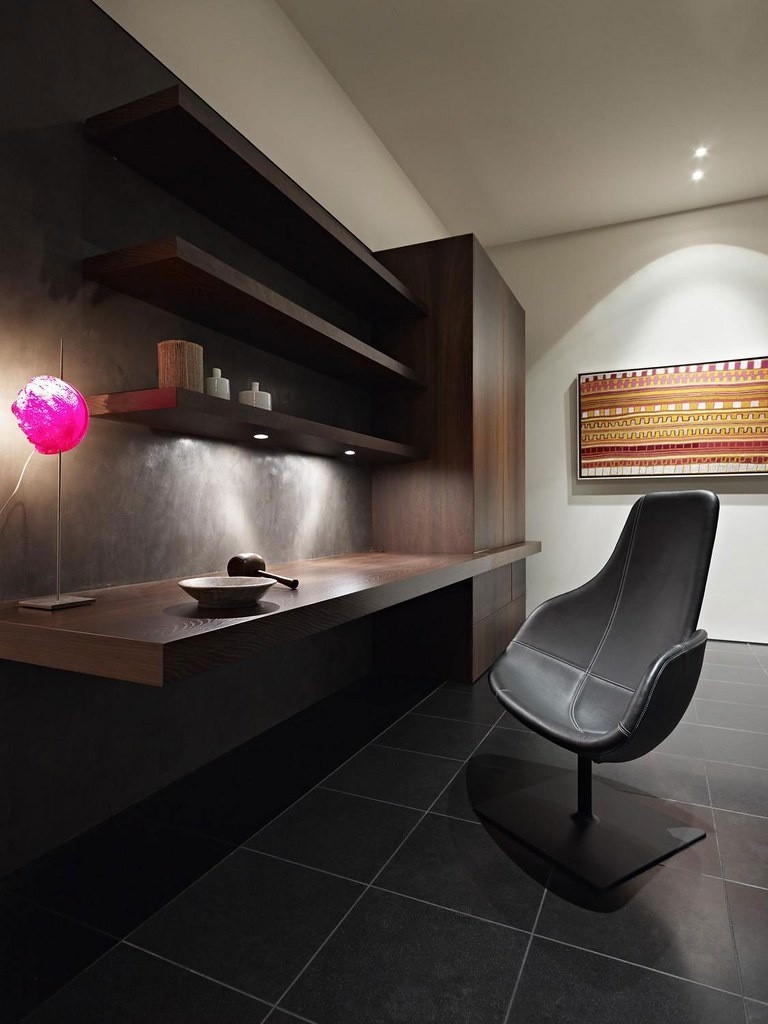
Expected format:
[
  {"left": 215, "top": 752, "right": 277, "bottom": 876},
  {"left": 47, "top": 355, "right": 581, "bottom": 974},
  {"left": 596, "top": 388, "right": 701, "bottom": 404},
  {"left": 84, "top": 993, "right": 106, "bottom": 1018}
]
[{"left": 0, "top": 0, "right": 540, "bottom": 691}]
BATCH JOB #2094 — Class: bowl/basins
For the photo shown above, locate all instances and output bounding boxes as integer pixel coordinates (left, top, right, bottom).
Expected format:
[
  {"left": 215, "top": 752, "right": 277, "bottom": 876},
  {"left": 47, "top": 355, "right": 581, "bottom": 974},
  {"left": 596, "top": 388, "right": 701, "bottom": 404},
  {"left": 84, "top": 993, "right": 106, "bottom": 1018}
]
[{"left": 179, "top": 576, "right": 277, "bottom": 607}]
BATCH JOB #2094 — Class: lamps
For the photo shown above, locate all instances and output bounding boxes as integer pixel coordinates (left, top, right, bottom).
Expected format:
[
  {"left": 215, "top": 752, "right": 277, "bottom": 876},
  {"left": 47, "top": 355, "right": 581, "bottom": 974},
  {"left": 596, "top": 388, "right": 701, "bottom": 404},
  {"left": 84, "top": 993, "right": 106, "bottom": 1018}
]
[{"left": 11, "top": 339, "right": 97, "bottom": 611}]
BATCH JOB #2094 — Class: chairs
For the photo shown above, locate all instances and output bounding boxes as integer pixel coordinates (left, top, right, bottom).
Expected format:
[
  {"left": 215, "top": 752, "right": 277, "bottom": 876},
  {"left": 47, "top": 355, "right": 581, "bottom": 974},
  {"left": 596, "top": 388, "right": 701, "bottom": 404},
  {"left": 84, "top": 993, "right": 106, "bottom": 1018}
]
[{"left": 465, "top": 492, "right": 720, "bottom": 894}]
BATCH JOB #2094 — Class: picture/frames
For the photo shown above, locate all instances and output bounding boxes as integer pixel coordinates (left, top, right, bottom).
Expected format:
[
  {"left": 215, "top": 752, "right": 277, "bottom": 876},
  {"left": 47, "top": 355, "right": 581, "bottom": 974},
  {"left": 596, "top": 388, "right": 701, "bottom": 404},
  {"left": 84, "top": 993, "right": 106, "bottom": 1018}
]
[{"left": 576, "top": 357, "right": 768, "bottom": 478}]
